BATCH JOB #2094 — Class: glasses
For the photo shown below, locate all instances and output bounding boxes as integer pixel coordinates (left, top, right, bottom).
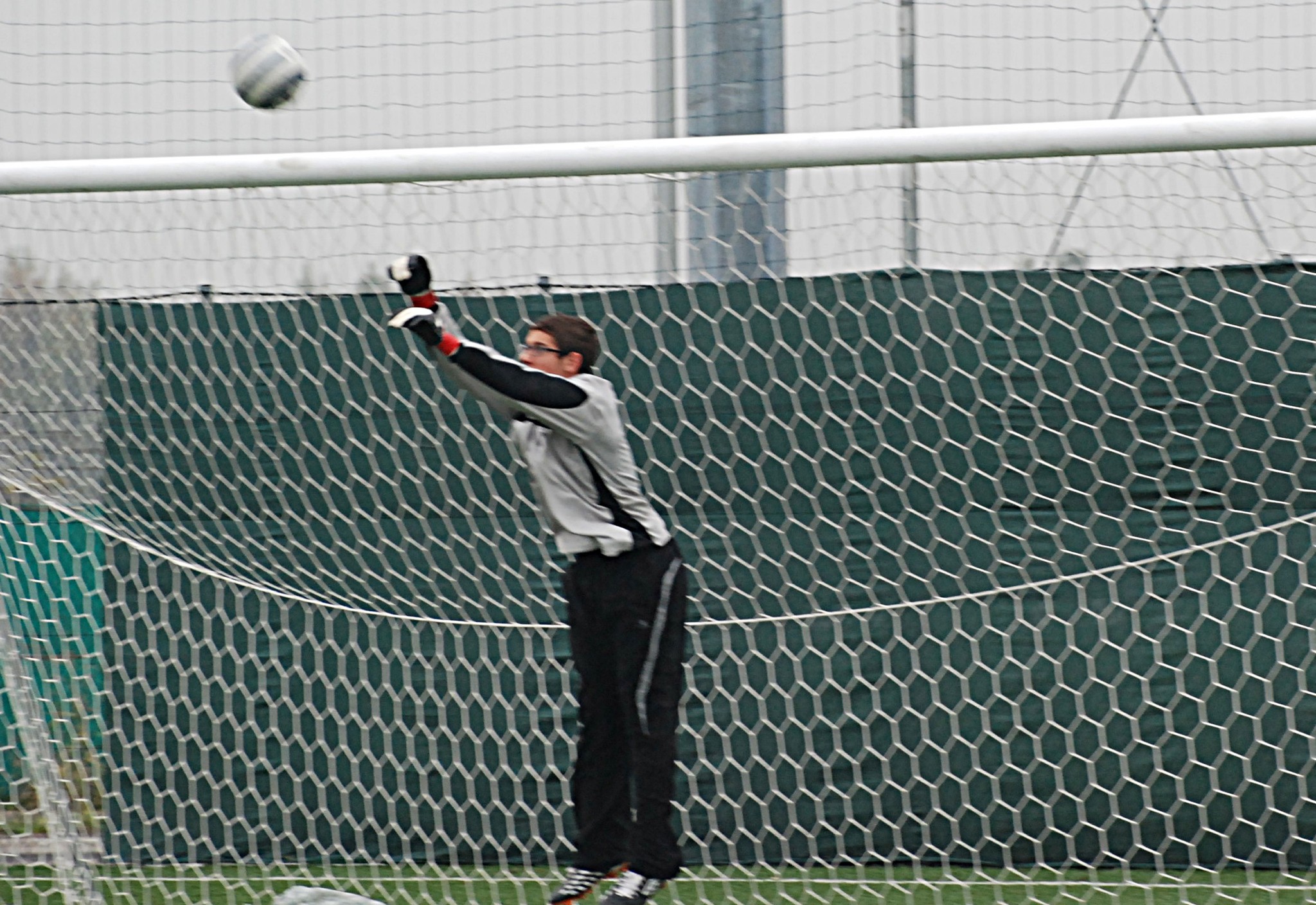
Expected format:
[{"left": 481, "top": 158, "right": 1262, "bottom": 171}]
[{"left": 515, "top": 343, "right": 564, "bottom": 357}]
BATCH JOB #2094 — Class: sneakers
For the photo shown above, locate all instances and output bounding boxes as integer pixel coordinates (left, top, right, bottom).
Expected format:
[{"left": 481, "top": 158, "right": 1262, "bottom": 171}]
[
  {"left": 604, "top": 869, "right": 668, "bottom": 905},
  {"left": 549, "top": 863, "right": 628, "bottom": 905}
]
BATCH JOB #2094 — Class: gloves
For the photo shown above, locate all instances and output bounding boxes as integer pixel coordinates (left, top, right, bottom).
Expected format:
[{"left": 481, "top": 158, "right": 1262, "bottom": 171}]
[
  {"left": 386, "top": 254, "right": 438, "bottom": 310},
  {"left": 386, "top": 305, "right": 458, "bottom": 355}
]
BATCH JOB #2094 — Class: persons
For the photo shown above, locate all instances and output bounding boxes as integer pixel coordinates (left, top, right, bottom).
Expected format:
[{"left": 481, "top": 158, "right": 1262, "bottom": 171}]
[{"left": 387, "top": 255, "right": 688, "bottom": 905}]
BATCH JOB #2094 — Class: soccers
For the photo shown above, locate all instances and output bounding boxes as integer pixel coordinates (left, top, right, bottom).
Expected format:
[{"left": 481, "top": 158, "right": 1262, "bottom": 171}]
[{"left": 231, "top": 34, "right": 307, "bottom": 109}]
